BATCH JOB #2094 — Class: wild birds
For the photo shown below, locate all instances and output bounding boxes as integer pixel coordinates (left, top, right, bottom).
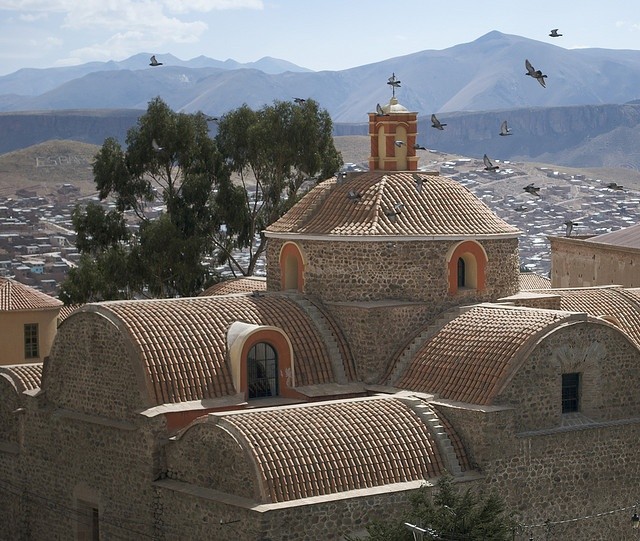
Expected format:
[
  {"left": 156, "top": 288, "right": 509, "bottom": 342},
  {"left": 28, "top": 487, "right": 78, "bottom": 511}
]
[
  {"left": 431, "top": 114, "right": 447, "bottom": 130},
  {"left": 514, "top": 205, "right": 527, "bottom": 212},
  {"left": 562, "top": 220, "right": 578, "bottom": 238},
  {"left": 394, "top": 141, "right": 404, "bottom": 147},
  {"left": 385, "top": 209, "right": 401, "bottom": 223},
  {"left": 150, "top": 138, "right": 164, "bottom": 154},
  {"left": 292, "top": 97, "right": 305, "bottom": 102},
  {"left": 202, "top": 114, "right": 218, "bottom": 122},
  {"left": 394, "top": 203, "right": 405, "bottom": 210},
  {"left": 522, "top": 183, "right": 540, "bottom": 196},
  {"left": 607, "top": 182, "right": 623, "bottom": 191},
  {"left": 499, "top": 120, "right": 513, "bottom": 136},
  {"left": 483, "top": 154, "right": 499, "bottom": 172},
  {"left": 525, "top": 59, "right": 548, "bottom": 89},
  {"left": 549, "top": 28, "right": 562, "bottom": 38},
  {"left": 411, "top": 173, "right": 428, "bottom": 193},
  {"left": 387, "top": 76, "right": 401, "bottom": 87},
  {"left": 149, "top": 55, "right": 163, "bottom": 66},
  {"left": 347, "top": 191, "right": 363, "bottom": 204},
  {"left": 412, "top": 144, "right": 426, "bottom": 150},
  {"left": 376, "top": 103, "right": 390, "bottom": 116}
]
